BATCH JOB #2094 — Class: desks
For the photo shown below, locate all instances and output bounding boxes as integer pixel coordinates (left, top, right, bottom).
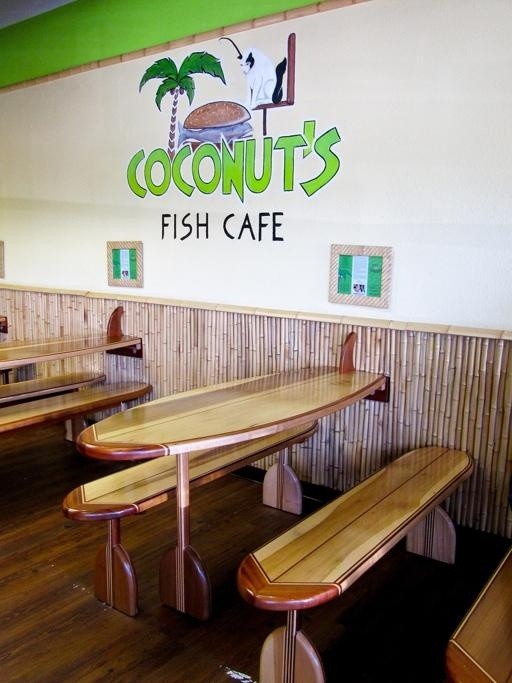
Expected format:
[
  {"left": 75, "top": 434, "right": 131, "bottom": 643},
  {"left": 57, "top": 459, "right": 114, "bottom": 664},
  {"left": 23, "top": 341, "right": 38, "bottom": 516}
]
[{"left": 0, "top": 331, "right": 143, "bottom": 372}]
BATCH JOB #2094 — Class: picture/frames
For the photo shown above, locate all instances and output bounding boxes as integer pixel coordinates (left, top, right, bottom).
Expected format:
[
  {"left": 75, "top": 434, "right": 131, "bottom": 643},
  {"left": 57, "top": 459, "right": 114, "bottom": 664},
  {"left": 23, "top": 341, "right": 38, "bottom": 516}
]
[
  {"left": 328, "top": 243, "right": 392, "bottom": 311},
  {"left": 107, "top": 240, "right": 144, "bottom": 288}
]
